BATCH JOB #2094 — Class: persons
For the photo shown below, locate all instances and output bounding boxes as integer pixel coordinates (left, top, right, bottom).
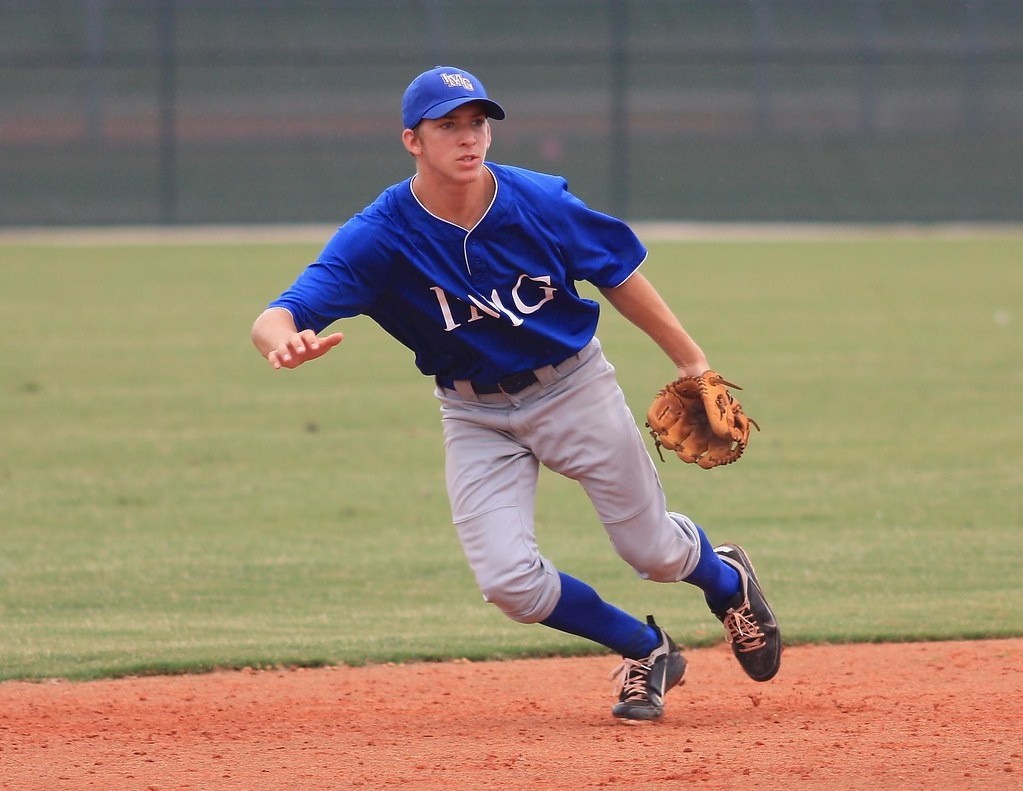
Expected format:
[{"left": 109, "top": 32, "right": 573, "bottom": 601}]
[{"left": 250, "top": 66, "right": 782, "bottom": 724}]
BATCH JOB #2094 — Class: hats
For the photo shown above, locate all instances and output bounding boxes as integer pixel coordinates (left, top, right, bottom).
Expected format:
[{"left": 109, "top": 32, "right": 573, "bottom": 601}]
[{"left": 403, "top": 65, "right": 506, "bottom": 128}]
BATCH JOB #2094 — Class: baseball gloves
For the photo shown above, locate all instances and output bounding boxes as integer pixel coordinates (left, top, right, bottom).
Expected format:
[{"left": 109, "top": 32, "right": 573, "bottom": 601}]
[{"left": 645, "top": 369, "right": 752, "bottom": 470}]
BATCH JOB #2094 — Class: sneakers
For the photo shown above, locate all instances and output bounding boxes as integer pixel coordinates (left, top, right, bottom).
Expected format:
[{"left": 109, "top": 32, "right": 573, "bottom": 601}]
[
  {"left": 709, "top": 542, "right": 782, "bottom": 682},
  {"left": 607, "top": 615, "right": 689, "bottom": 724}
]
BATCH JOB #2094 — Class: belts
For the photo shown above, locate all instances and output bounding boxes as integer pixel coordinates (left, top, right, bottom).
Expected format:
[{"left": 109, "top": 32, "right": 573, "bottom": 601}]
[{"left": 440, "top": 360, "right": 559, "bottom": 394}]
